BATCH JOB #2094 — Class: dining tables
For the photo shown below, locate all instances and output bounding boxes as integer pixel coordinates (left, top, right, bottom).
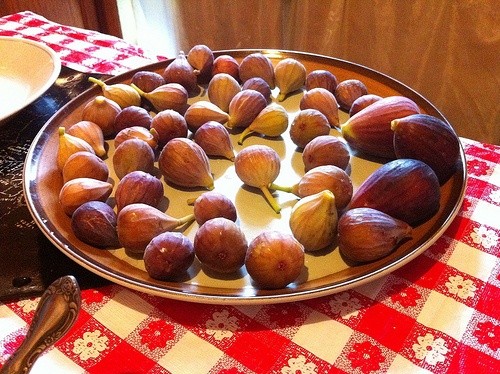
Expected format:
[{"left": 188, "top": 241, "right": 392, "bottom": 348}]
[{"left": 0, "top": 9, "right": 500, "bottom": 374}]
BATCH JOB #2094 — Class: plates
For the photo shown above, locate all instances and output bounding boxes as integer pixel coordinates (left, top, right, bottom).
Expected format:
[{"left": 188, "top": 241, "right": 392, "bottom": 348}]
[
  {"left": 20, "top": 49, "right": 468, "bottom": 304},
  {"left": 0, "top": 36, "right": 62, "bottom": 126}
]
